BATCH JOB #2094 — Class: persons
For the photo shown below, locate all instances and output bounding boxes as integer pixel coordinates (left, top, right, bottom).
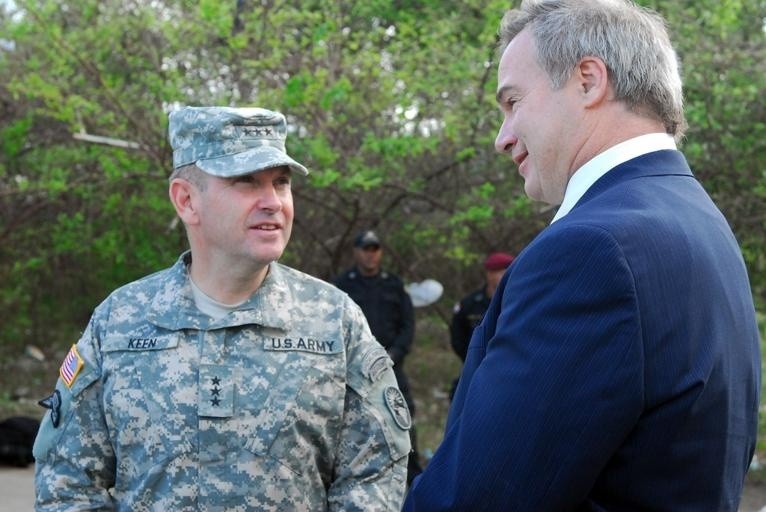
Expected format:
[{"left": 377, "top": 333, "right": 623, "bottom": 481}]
[
  {"left": 398, "top": 0, "right": 764, "bottom": 510},
  {"left": 28, "top": 104, "right": 412, "bottom": 511},
  {"left": 328, "top": 226, "right": 426, "bottom": 490},
  {"left": 450, "top": 253, "right": 515, "bottom": 401}
]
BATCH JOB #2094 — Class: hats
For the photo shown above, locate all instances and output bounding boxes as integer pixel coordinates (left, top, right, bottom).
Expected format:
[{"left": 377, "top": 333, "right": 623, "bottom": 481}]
[
  {"left": 352, "top": 230, "right": 383, "bottom": 251},
  {"left": 168, "top": 105, "right": 310, "bottom": 181},
  {"left": 483, "top": 251, "right": 515, "bottom": 272}
]
